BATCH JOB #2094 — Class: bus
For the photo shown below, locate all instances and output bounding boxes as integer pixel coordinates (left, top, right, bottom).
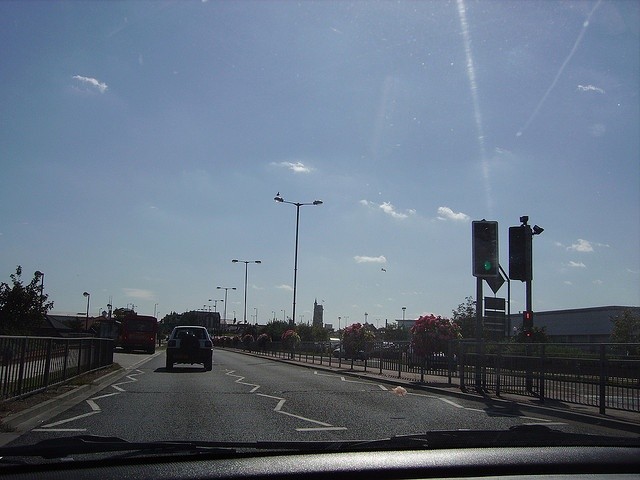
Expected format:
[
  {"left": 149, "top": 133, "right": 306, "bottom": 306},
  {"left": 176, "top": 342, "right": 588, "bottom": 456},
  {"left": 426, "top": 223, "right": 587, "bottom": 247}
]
[{"left": 121, "top": 316, "right": 157, "bottom": 354}]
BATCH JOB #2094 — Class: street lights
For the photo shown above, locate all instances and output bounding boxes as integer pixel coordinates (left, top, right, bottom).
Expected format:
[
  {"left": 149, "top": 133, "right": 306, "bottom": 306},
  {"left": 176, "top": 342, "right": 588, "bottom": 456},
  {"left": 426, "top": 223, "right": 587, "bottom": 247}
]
[
  {"left": 107, "top": 304, "right": 112, "bottom": 321},
  {"left": 209, "top": 299, "right": 224, "bottom": 339},
  {"left": 274, "top": 192, "right": 323, "bottom": 360},
  {"left": 217, "top": 287, "right": 236, "bottom": 348},
  {"left": 232, "top": 260, "right": 261, "bottom": 351},
  {"left": 83, "top": 292, "right": 90, "bottom": 364},
  {"left": 34, "top": 271, "right": 44, "bottom": 314}
]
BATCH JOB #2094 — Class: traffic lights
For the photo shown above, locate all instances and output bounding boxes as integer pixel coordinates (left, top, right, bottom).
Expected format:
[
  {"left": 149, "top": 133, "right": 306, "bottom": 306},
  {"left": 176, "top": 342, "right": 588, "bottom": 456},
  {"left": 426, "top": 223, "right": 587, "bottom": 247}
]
[
  {"left": 525, "top": 331, "right": 532, "bottom": 339},
  {"left": 523, "top": 311, "right": 533, "bottom": 330},
  {"left": 509, "top": 226, "right": 532, "bottom": 280},
  {"left": 472, "top": 220, "right": 499, "bottom": 277}
]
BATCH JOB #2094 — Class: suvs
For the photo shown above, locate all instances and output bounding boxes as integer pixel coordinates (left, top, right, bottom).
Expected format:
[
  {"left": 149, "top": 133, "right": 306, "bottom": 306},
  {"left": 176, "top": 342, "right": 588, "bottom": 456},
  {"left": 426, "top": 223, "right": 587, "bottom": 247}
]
[{"left": 166, "top": 326, "right": 214, "bottom": 371}]
